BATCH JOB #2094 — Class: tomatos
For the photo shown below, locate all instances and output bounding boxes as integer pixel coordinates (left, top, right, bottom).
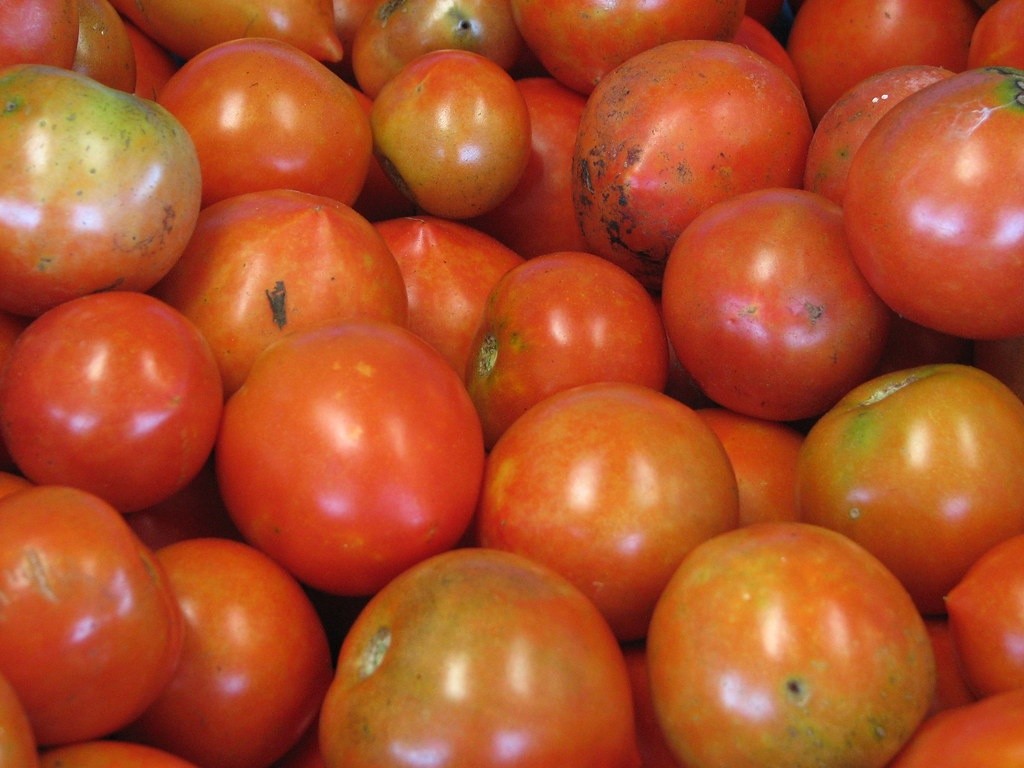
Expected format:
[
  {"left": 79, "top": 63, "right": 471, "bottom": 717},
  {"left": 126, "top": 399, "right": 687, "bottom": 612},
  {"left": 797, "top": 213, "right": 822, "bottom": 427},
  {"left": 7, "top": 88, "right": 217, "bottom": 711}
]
[{"left": 0, "top": 0, "right": 1024, "bottom": 768}]
